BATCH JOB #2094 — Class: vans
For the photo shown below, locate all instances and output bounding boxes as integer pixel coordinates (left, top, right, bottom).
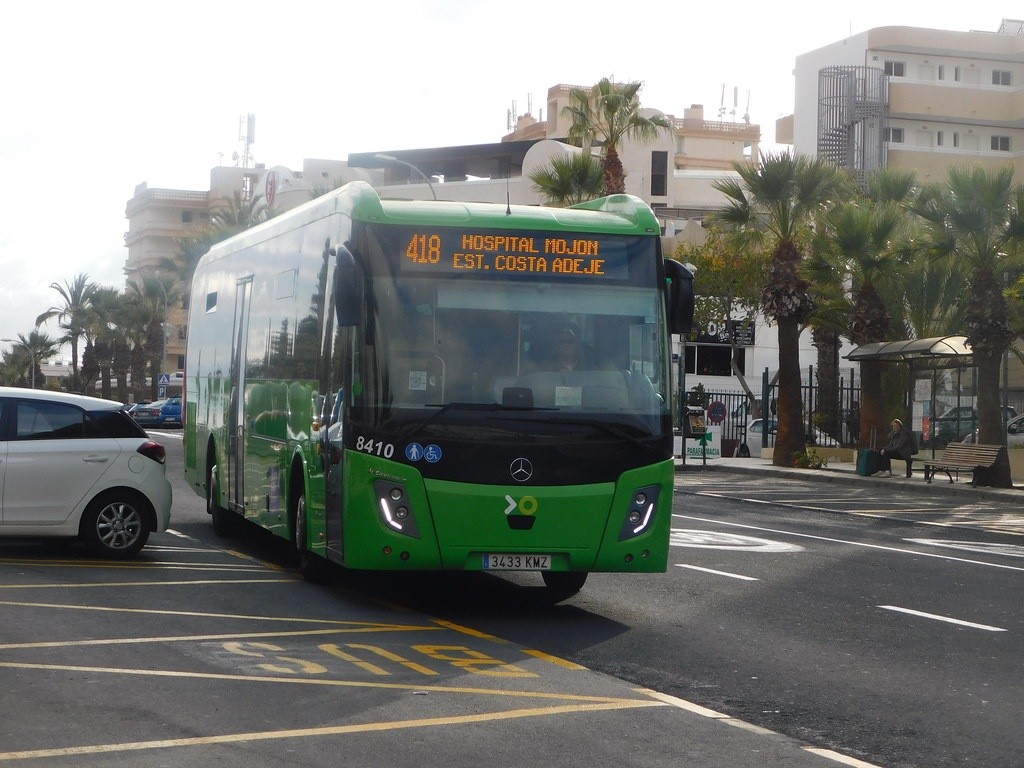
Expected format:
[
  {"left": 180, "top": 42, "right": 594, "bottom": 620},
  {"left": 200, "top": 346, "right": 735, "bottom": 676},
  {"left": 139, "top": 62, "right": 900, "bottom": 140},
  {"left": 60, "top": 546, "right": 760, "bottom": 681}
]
[{"left": 730, "top": 396, "right": 779, "bottom": 434}]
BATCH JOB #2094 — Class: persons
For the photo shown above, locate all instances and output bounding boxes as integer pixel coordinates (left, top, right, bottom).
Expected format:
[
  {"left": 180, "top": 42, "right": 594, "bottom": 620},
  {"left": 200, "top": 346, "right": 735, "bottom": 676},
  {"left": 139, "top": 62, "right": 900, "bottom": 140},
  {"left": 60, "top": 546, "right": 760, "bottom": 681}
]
[
  {"left": 871, "top": 419, "right": 917, "bottom": 477},
  {"left": 546, "top": 329, "right": 585, "bottom": 371},
  {"left": 847, "top": 401, "right": 861, "bottom": 443}
]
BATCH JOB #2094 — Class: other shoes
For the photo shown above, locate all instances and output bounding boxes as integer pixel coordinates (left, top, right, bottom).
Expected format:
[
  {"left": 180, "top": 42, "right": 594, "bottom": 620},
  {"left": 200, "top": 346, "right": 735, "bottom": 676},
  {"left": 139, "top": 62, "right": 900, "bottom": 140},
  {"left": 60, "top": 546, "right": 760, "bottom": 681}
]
[{"left": 871, "top": 471, "right": 891, "bottom": 478}]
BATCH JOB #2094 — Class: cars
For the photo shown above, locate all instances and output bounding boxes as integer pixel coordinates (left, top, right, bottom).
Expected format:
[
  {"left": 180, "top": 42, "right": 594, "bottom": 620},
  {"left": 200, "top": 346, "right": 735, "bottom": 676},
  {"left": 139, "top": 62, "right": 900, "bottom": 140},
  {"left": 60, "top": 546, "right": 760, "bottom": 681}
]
[
  {"left": 939, "top": 404, "right": 1024, "bottom": 449},
  {"left": 124, "top": 397, "right": 183, "bottom": 427},
  {"left": 0, "top": 385, "right": 173, "bottom": 559},
  {"left": 736, "top": 418, "right": 842, "bottom": 458}
]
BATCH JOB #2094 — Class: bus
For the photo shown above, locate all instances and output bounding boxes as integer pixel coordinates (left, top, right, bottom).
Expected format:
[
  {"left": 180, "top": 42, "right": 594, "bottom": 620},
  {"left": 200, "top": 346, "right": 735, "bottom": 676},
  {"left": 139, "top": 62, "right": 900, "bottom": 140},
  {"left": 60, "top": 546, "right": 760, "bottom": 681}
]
[{"left": 179, "top": 178, "right": 698, "bottom": 601}]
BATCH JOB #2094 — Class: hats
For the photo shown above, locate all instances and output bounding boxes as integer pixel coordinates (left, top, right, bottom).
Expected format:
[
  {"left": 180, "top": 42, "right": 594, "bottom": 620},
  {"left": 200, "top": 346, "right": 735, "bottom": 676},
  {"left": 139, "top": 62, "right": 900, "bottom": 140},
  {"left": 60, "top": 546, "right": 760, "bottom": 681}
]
[{"left": 553, "top": 322, "right": 581, "bottom": 341}]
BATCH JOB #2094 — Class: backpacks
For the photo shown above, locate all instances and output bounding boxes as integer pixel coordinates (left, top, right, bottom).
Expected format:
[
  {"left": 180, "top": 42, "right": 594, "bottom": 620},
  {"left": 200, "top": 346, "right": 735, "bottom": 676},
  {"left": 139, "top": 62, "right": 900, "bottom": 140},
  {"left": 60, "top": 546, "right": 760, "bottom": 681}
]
[{"left": 911, "top": 437, "right": 918, "bottom": 455}]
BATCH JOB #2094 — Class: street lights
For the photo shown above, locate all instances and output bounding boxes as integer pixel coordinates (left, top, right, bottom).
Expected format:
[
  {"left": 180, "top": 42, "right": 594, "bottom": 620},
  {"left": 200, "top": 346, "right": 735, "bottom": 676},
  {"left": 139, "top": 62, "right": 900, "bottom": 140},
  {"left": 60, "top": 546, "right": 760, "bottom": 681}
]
[
  {"left": 1, "top": 338, "right": 35, "bottom": 388},
  {"left": 123, "top": 266, "right": 168, "bottom": 373}
]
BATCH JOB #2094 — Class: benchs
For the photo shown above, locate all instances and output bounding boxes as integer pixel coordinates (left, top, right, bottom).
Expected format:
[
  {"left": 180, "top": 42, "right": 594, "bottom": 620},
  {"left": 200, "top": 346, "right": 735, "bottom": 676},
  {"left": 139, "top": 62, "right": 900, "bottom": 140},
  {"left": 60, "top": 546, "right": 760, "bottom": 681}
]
[
  {"left": 922, "top": 441, "right": 1004, "bottom": 488},
  {"left": 876, "top": 454, "right": 930, "bottom": 480}
]
